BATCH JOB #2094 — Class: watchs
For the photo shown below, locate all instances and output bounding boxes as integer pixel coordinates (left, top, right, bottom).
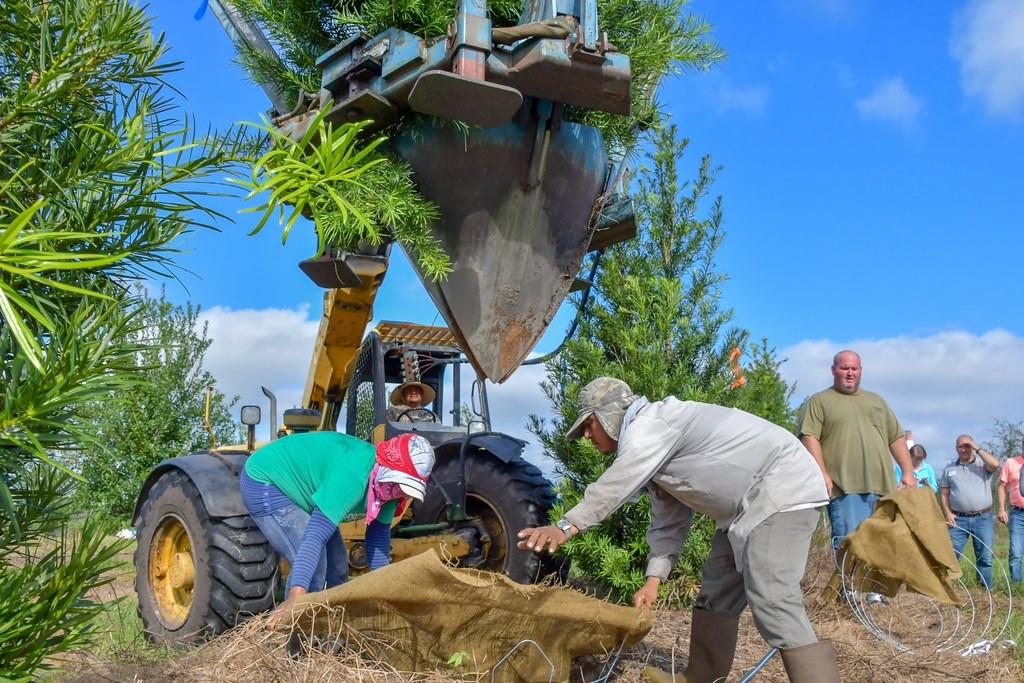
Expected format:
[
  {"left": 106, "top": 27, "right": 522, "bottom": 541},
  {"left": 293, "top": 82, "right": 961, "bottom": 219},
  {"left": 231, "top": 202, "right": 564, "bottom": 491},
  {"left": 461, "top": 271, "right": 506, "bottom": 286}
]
[{"left": 557, "top": 519, "right": 572, "bottom": 538}]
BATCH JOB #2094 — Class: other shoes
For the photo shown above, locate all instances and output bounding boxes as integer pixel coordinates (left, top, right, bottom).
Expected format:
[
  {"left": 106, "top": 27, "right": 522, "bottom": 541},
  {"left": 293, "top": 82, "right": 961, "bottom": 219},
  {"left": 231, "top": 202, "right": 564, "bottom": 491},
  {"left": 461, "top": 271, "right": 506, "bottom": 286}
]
[
  {"left": 316, "top": 632, "right": 347, "bottom": 656},
  {"left": 286, "top": 636, "right": 305, "bottom": 660},
  {"left": 838, "top": 588, "right": 862, "bottom": 608},
  {"left": 867, "top": 591, "right": 892, "bottom": 606}
]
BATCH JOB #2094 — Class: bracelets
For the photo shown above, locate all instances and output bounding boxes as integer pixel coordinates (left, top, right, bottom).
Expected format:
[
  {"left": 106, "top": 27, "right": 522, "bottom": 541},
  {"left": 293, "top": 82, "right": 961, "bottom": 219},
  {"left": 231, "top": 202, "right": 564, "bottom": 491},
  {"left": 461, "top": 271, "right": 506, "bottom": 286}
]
[{"left": 976, "top": 448, "right": 982, "bottom": 453}]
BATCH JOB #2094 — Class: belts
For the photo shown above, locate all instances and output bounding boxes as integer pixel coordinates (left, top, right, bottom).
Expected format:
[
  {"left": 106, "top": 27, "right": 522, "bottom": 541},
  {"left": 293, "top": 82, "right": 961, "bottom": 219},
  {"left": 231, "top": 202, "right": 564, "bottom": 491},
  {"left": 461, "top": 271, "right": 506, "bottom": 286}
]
[
  {"left": 1014, "top": 506, "right": 1024, "bottom": 512},
  {"left": 951, "top": 506, "right": 992, "bottom": 517}
]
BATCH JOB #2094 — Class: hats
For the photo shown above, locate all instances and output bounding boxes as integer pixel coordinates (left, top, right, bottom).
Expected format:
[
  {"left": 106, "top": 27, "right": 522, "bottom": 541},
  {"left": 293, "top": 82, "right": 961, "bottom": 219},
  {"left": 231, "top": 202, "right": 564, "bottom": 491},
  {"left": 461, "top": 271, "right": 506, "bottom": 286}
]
[
  {"left": 398, "top": 433, "right": 436, "bottom": 503},
  {"left": 564, "top": 377, "right": 641, "bottom": 442},
  {"left": 389, "top": 381, "right": 436, "bottom": 407}
]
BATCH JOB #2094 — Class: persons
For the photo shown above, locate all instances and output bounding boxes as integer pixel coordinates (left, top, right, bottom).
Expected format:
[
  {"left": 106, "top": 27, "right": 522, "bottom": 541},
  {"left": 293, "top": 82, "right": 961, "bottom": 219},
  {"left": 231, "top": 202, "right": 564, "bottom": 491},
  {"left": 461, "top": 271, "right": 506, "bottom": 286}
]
[
  {"left": 941, "top": 435, "right": 1000, "bottom": 591},
  {"left": 997, "top": 440, "right": 1024, "bottom": 582},
  {"left": 895, "top": 444, "right": 938, "bottom": 495},
  {"left": 517, "top": 376, "right": 841, "bottom": 683},
  {"left": 386, "top": 382, "right": 441, "bottom": 423},
  {"left": 796, "top": 350, "right": 916, "bottom": 605},
  {"left": 239, "top": 431, "right": 436, "bottom": 653}
]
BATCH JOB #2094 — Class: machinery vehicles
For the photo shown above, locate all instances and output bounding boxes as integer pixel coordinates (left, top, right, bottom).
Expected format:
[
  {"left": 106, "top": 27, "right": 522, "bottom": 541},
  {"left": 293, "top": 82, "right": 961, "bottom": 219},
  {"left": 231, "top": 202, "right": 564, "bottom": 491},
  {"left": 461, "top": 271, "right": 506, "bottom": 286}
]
[{"left": 129, "top": 0, "right": 634, "bottom": 652}]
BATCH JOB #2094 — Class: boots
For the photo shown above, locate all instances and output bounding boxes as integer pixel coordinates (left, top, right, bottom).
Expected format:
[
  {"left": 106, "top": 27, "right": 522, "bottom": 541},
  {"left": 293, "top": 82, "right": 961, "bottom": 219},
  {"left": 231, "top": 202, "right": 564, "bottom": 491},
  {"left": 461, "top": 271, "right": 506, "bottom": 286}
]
[
  {"left": 639, "top": 607, "right": 739, "bottom": 683},
  {"left": 780, "top": 638, "right": 841, "bottom": 682}
]
